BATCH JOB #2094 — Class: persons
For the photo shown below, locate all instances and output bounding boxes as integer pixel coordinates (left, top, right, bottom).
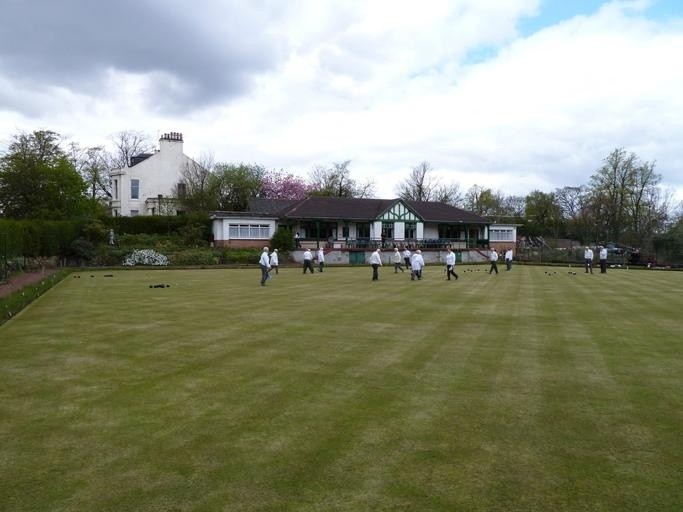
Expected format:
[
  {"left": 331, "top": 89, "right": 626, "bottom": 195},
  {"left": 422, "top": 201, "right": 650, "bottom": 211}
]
[
  {"left": 108, "top": 228, "right": 115, "bottom": 245},
  {"left": 584, "top": 247, "right": 594, "bottom": 274},
  {"left": 446, "top": 248, "right": 458, "bottom": 281},
  {"left": 318, "top": 247, "right": 325, "bottom": 272},
  {"left": 488, "top": 248, "right": 499, "bottom": 274},
  {"left": 411, "top": 250, "right": 425, "bottom": 280},
  {"left": 402, "top": 248, "right": 411, "bottom": 269},
  {"left": 393, "top": 248, "right": 404, "bottom": 273},
  {"left": 599, "top": 246, "right": 607, "bottom": 274},
  {"left": 295, "top": 232, "right": 302, "bottom": 248},
  {"left": 268, "top": 249, "right": 278, "bottom": 274},
  {"left": 505, "top": 248, "right": 513, "bottom": 271},
  {"left": 303, "top": 248, "right": 314, "bottom": 274},
  {"left": 369, "top": 249, "right": 382, "bottom": 281},
  {"left": 258, "top": 247, "right": 271, "bottom": 288}
]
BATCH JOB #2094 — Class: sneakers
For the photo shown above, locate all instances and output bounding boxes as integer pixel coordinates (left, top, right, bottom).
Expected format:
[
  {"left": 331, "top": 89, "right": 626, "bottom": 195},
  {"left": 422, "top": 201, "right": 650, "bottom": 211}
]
[
  {"left": 488, "top": 272, "right": 492, "bottom": 274},
  {"left": 506, "top": 269, "right": 513, "bottom": 272},
  {"left": 261, "top": 281, "right": 265, "bottom": 286},
  {"left": 455, "top": 275, "right": 458, "bottom": 280},
  {"left": 446, "top": 278, "right": 451, "bottom": 280},
  {"left": 276, "top": 272, "right": 278, "bottom": 275},
  {"left": 496, "top": 271, "right": 499, "bottom": 274},
  {"left": 303, "top": 270, "right": 323, "bottom": 275},
  {"left": 402, "top": 266, "right": 412, "bottom": 273},
  {"left": 395, "top": 272, "right": 397, "bottom": 273},
  {"left": 418, "top": 278, "right": 422, "bottom": 280},
  {"left": 411, "top": 278, "right": 415, "bottom": 280},
  {"left": 372, "top": 277, "right": 379, "bottom": 280}
]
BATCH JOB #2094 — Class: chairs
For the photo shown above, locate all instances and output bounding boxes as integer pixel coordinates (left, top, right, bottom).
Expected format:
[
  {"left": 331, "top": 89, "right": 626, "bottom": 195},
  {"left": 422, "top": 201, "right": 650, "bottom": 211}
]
[{"left": 331, "top": 238, "right": 463, "bottom": 250}]
